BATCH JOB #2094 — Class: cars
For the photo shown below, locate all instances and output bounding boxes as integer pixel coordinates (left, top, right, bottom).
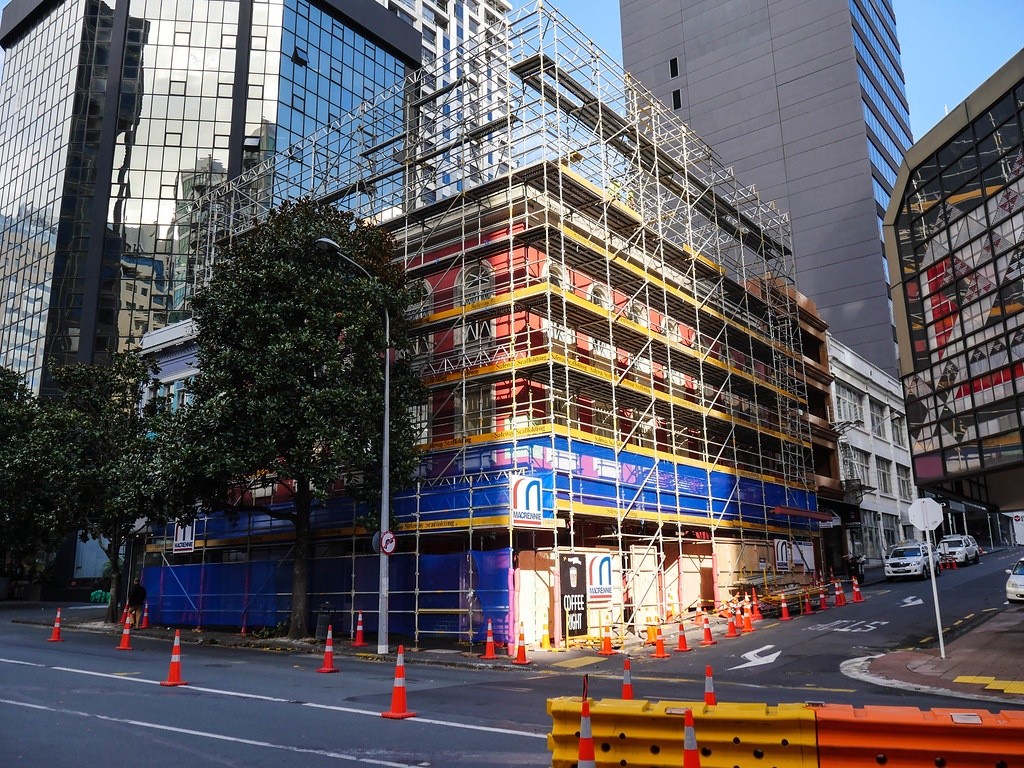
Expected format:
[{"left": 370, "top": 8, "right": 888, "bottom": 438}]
[{"left": 1005, "top": 554, "right": 1024, "bottom": 605}]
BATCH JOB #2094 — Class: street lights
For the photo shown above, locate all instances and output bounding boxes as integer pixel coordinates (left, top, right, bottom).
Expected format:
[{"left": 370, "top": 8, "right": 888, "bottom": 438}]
[{"left": 313, "top": 236, "right": 392, "bottom": 655}]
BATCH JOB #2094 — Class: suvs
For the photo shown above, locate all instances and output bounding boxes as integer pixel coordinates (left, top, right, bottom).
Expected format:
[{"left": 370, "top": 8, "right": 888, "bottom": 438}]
[
  {"left": 883, "top": 539, "right": 942, "bottom": 584},
  {"left": 936, "top": 533, "right": 980, "bottom": 567}
]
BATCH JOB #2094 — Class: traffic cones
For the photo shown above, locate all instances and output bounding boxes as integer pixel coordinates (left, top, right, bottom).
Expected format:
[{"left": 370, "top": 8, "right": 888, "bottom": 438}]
[
  {"left": 946, "top": 558, "right": 951, "bottom": 570},
  {"left": 723, "top": 604, "right": 742, "bottom": 638},
  {"left": 380, "top": 644, "right": 417, "bottom": 720},
  {"left": 577, "top": 701, "right": 597, "bottom": 768},
  {"left": 649, "top": 617, "right": 671, "bottom": 659},
  {"left": 315, "top": 624, "right": 340, "bottom": 673},
  {"left": 511, "top": 621, "right": 532, "bottom": 666},
  {"left": 733, "top": 587, "right": 764, "bottom": 634},
  {"left": 350, "top": 611, "right": 369, "bottom": 648},
  {"left": 117, "top": 604, "right": 129, "bottom": 625},
  {"left": 534, "top": 614, "right": 554, "bottom": 651},
  {"left": 672, "top": 614, "right": 692, "bottom": 653},
  {"left": 682, "top": 709, "right": 701, "bottom": 768},
  {"left": 620, "top": 658, "right": 634, "bottom": 700},
  {"left": 160, "top": 629, "right": 188, "bottom": 686},
  {"left": 817, "top": 581, "right": 830, "bottom": 610},
  {"left": 703, "top": 664, "right": 717, "bottom": 705},
  {"left": 115, "top": 612, "right": 134, "bottom": 650},
  {"left": 939, "top": 559, "right": 944, "bottom": 570},
  {"left": 643, "top": 607, "right": 659, "bottom": 646},
  {"left": 778, "top": 588, "right": 793, "bottom": 621},
  {"left": 802, "top": 587, "right": 816, "bottom": 615},
  {"left": 46, "top": 607, "right": 64, "bottom": 642},
  {"left": 596, "top": 614, "right": 618, "bottom": 655},
  {"left": 138, "top": 601, "right": 151, "bottom": 629},
  {"left": 662, "top": 597, "right": 677, "bottom": 624},
  {"left": 478, "top": 618, "right": 501, "bottom": 660},
  {"left": 851, "top": 575, "right": 865, "bottom": 603},
  {"left": 699, "top": 607, "right": 718, "bottom": 645},
  {"left": 834, "top": 578, "right": 849, "bottom": 607},
  {"left": 691, "top": 594, "right": 704, "bottom": 626},
  {"left": 952, "top": 556, "right": 959, "bottom": 570}
]
